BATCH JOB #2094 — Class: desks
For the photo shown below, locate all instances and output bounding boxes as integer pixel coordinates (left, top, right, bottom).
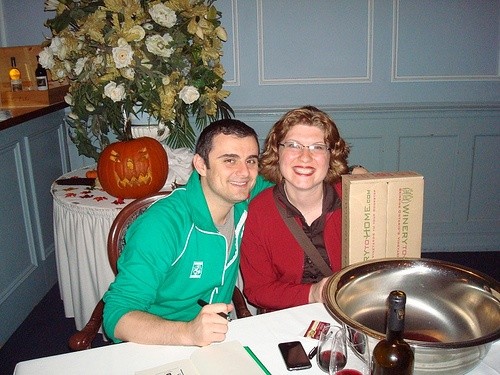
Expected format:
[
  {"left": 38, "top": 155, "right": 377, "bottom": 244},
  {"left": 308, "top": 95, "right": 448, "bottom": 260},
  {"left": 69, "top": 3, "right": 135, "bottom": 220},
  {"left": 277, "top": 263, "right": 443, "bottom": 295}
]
[
  {"left": 48, "top": 156, "right": 259, "bottom": 341},
  {"left": 9, "top": 299, "right": 500, "bottom": 375}
]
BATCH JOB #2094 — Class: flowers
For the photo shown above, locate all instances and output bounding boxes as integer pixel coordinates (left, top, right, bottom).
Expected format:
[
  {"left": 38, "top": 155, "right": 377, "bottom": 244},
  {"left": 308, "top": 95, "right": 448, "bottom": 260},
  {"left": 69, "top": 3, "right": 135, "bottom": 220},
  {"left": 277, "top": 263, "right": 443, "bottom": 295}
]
[{"left": 36, "top": 0, "right": 235, "bottom": 157}]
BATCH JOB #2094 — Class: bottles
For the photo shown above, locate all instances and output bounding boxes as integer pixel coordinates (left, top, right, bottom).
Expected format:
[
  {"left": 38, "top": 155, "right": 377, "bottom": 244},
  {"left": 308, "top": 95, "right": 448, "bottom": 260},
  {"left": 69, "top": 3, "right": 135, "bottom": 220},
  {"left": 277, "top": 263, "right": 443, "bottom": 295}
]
[
  {"left": 35, "top": 54, "right": 49, "bottom": 91},
  {"left": 372, "top": 290, "right": 414, "bottom": 374},
  {"left": 9, "top": 56, "right": 22, "bottom": 92}
]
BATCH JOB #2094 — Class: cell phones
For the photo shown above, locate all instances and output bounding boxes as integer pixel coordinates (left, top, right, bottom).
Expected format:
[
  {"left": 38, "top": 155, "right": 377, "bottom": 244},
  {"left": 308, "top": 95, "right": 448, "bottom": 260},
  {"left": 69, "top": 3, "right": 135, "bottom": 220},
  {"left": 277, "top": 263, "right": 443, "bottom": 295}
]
[{"left": 279, "top": 341, "right": 312, "bottom": 371}]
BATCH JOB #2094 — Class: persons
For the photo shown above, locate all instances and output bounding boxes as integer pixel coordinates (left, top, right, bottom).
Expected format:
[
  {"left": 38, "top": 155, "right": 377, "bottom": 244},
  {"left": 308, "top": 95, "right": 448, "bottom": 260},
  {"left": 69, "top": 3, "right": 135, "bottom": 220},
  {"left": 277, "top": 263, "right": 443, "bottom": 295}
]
[
  {"left": 103, "top": 118, "right": 261, "bottom": 346},
  {"left": 239, "top": 107, "right": 371, "bottom": 315}
]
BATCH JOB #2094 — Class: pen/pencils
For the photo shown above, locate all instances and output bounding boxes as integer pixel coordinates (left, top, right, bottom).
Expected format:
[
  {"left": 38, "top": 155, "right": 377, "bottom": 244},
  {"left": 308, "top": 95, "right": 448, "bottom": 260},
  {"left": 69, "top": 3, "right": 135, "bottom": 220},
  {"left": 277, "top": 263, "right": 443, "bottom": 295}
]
[
  {"left": 308, "top": 331, "right": 333, "bottom": 359},
  {"left": 197, "top": 299, "right": 232, "bottom": 322}
]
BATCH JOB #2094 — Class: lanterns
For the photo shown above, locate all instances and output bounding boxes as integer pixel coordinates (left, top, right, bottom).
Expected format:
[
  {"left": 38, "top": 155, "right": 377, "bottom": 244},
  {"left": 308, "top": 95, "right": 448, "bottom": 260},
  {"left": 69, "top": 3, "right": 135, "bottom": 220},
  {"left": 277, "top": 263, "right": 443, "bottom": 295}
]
[{"left": 97, "top": 136, "right": 169, "bottom": 197}]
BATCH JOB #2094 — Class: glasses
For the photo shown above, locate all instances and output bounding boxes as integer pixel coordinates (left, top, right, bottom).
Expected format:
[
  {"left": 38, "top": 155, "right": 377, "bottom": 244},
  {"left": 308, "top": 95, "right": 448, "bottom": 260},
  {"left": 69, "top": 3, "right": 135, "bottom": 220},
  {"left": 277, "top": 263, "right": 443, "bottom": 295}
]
[{"left": 276, "top": 141, "right": 332, "bottom": 156}]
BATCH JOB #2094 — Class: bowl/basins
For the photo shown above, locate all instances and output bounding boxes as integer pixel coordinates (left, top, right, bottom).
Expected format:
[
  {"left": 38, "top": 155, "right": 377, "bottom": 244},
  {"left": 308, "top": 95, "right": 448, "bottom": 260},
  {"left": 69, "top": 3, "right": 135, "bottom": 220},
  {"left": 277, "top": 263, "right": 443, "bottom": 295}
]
[{"left": 320, "top": 256, "right": 500, "bottom": 375}]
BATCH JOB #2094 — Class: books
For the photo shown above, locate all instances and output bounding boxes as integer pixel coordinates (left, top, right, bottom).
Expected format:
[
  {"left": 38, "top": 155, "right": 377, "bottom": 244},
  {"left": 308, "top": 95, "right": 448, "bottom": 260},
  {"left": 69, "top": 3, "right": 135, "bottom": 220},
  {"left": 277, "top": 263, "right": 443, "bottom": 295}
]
[{"left": 135, "top": 341, "right": 273, "bottom": 375}]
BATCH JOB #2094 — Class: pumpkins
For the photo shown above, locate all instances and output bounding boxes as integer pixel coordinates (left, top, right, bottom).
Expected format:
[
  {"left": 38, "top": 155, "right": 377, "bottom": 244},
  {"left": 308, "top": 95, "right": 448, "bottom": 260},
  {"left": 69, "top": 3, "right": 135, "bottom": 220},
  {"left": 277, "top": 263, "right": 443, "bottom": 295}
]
[{"left": 98, "top": 120, "right": 168, "bottom": 199}]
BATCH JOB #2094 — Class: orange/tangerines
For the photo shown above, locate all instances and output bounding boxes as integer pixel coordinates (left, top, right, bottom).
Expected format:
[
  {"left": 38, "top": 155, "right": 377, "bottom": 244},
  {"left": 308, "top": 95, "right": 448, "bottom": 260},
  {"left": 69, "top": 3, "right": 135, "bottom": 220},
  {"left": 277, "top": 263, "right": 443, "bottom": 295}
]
[{"left": 86, "top": 168, "right": 98, "bottom": 178}]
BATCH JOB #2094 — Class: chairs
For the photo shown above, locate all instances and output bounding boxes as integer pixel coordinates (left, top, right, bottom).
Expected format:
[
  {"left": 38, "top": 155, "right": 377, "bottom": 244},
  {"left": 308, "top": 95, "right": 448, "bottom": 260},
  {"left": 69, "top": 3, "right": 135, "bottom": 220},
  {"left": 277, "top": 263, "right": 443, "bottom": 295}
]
[{"left": 67, "top": 191, "right": 253, "bottom": 352}]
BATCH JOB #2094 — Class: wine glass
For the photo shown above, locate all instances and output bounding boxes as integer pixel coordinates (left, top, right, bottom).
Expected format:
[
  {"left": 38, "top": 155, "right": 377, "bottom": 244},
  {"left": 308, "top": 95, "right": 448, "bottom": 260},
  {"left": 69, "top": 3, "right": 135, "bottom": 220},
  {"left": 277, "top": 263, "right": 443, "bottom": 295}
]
[
  {"left": 315, "top": 325, "right": 348, "bottom": 375},
  {"left": 327, "top": 324, "right": 372, "bottom": 375}
]
